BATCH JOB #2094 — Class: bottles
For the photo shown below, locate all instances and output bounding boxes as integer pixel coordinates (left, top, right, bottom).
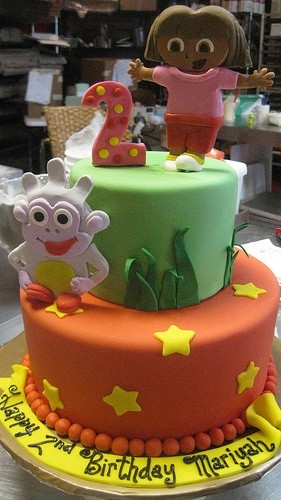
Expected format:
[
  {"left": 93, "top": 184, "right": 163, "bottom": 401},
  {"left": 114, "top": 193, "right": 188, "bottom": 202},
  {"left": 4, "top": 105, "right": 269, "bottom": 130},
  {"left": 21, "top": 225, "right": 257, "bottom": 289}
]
[
  {"left": 248, "top": 112, "right": 255, "bottom": 126},
  {"left": 136, "top": 27, "right": 144, "bottom": 48}
]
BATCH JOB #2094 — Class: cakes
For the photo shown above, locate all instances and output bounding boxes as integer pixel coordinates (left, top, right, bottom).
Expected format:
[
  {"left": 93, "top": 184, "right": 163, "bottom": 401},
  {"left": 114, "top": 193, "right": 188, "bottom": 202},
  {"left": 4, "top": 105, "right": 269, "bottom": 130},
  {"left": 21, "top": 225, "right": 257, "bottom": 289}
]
[{"left": 0, "top": 6, "right": 281, "bottom": 489}]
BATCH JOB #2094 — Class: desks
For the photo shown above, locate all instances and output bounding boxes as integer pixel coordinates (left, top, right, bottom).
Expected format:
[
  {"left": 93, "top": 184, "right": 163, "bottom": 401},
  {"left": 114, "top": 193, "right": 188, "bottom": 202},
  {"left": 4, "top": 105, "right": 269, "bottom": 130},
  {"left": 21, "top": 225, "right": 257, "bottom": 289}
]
[{"left": 219, "top": 113, "right": 281, "bottom": 161}]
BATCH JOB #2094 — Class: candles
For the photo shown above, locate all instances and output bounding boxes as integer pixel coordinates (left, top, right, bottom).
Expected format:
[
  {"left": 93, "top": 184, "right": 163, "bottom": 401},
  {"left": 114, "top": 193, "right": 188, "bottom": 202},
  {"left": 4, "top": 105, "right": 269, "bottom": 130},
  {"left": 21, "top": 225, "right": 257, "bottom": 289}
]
[{"left": 80, "top": 80, "right": 148, "bottom": 165}]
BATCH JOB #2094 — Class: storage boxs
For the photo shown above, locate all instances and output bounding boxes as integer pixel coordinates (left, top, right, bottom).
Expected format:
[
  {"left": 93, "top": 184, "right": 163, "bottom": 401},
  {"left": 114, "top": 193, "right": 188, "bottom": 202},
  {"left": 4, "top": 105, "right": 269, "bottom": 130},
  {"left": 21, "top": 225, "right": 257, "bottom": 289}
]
[
  {"left": 26, "top": 57, "right": 140, "bottom": 120},
  {"left": 223, "top": 92, "right": 267, "bottom": 124}
]
